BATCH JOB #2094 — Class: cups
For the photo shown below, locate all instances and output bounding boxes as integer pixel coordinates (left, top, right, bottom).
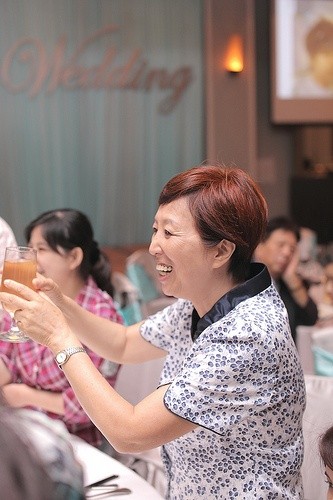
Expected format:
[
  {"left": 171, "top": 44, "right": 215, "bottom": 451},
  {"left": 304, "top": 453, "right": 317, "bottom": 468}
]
[{"left": 322, "top": 280, "right": 333, "bottom": 305}]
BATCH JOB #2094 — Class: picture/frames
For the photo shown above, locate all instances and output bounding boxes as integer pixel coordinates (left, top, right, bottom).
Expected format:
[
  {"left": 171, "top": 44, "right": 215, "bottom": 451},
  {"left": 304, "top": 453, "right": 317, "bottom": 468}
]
[{"left": 264, "top": 0, "right": 333, "bottom": 127}]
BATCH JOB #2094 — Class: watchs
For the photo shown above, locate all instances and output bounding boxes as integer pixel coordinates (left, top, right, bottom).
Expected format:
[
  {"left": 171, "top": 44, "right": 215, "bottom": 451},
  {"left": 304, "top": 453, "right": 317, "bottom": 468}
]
[{"left": 53, "top": 347, "right": 87, "bottom": 372}]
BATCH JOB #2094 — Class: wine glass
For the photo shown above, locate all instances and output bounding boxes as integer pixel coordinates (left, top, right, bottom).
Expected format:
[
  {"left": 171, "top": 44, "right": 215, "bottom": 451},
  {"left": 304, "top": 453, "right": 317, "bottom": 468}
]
[{"left": 0, "top": 246, "right": 38, "bottom": 344}]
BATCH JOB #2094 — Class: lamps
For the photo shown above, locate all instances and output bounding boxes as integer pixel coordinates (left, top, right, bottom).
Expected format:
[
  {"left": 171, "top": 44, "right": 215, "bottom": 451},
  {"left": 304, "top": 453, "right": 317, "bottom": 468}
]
[{"left": 224, "top": 32, "right": 244, "bottom": 72}]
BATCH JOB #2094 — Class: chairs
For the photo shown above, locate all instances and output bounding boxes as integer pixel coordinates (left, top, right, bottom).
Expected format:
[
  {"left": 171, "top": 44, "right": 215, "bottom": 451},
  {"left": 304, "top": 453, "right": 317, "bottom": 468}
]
[{"left": 110, "top": 249, "right": 162, "bottom": 327}]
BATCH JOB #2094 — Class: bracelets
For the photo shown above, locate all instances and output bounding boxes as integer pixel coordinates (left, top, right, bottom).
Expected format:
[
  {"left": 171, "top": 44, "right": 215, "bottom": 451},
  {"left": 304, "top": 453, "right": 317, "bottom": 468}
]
[{"left": 289, "top": 285, "right": 303, "bottom": 292}]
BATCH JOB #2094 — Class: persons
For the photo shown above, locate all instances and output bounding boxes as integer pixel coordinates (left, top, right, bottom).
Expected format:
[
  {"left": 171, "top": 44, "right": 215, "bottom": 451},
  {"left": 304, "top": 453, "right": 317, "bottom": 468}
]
[
  {"left": 0, "top": 165, "right": 307, "bottom": 500},
  {"left": 253, "top": 217, "right": 333, "bottom": 350},
  {"left": 318, "top": 427, "right": 333, "bottom": 500},
  {"left": 2, "top": 209, "right": 127, "bottom": 454},
  {"left": 0, "top": 214, "right": 21, "bottom": 289}
]
